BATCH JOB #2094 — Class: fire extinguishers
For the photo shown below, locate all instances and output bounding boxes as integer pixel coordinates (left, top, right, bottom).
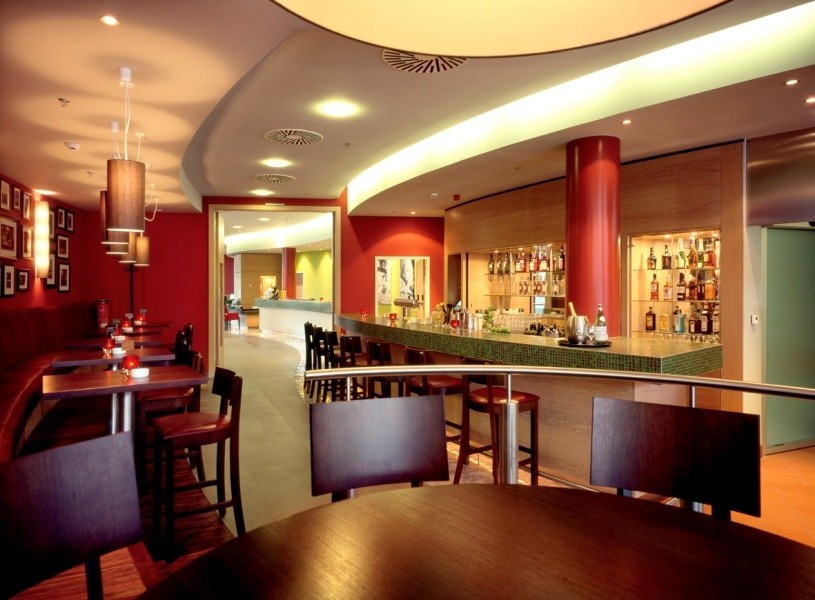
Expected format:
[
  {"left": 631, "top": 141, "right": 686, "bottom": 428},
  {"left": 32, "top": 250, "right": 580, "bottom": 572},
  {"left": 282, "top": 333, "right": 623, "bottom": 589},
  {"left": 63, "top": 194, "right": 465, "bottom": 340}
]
[{"left": 93, "top": 297, "right": 111, "bottom": 336}]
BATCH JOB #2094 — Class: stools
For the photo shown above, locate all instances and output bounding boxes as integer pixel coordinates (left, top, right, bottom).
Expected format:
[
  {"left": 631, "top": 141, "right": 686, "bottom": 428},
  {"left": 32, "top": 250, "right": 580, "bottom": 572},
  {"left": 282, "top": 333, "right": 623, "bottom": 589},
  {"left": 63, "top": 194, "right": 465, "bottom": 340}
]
[{"left": 137, "top": 321, "right": 540, "bottom": 567}]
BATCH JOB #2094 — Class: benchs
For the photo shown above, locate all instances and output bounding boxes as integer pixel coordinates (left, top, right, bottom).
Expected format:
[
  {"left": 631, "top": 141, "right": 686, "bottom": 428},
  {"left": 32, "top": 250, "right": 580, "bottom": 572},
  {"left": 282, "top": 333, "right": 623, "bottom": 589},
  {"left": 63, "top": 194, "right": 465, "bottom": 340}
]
[{"left": 0, "top": 298, "right": 115, "bottom": 463}]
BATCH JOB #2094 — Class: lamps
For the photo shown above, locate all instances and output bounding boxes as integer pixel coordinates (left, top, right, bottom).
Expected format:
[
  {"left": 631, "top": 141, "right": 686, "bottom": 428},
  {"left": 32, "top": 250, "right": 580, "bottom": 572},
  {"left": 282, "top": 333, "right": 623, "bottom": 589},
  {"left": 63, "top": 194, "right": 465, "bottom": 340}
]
[{"left": 98, "top": 67, "right": 156, "bottom": 267}]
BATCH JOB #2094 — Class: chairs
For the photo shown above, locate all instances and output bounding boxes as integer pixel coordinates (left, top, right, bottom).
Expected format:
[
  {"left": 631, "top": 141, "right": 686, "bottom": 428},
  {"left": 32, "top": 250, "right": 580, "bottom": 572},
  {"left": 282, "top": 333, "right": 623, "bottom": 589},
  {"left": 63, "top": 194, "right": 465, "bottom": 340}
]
[
  {"left": 309, "top": 394, "right": 449, "bottom": 513},
  {"left": 224, "top": 306, "right": 241, "bottom": 330},
  {"left": 0, "top": 428, "right": 138, "bottom": 600},
  {"left": 587, "top": 397, "right": 763, "bottom": 522}
]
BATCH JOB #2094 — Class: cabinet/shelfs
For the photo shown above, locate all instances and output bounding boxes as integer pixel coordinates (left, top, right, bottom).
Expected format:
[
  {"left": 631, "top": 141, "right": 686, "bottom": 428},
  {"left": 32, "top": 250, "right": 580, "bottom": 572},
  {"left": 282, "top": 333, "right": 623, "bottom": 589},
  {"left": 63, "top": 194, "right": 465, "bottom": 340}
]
[
  {"left": 630, "top": 229, "right": 720, "bottom": 343},
  {"left": 481, "top": 244, "right": 566, "bottom": 322}
]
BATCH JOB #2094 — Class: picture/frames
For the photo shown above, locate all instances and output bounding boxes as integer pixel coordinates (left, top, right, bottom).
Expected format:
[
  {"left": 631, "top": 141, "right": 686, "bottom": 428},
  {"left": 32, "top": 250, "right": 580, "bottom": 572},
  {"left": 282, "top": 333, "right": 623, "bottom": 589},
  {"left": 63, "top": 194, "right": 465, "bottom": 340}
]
[{"left": 0, "top": 177, "right": 75, "bottom": 298}]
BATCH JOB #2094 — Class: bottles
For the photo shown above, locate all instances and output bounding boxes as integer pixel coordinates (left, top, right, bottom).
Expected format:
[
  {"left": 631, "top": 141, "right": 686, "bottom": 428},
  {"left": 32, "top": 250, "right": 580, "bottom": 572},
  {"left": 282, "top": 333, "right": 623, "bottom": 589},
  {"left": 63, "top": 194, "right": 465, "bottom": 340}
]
[
  {"left": 676, "top": 273, "right": 689, "bottom": 300},
  {"left": 646, "top": 247, "right": 657, "bottom": 269},
  {"left": 650, "top": 274, "right": 659, "bottom": 300},
  {"left": 593, "top": 304, "right": 609, "bottom": 343},
  {"left": 703, "top": 237, "right": 720, "bottom": 269},
  {"left": 567, "top": 301, "right": 577, "bottom": 316},
  {"left": 673, "top": 306, "right": 681, "bottom": 330},
  {"left": 687, "top": 237, "right": 699, "bottom": 269},
  {"left": 689, "top": 304, "right": 720, "bottom": 334},
  {"left": 675, "top": 238, "right": 687, "bottom": 269},
  {"left": 661, "top": 243, "right": 672, "bottom": 269},
  {"left": 663, "top": 274, "right": 672, "bottom": 300},
  {"left": 659, "top": 309, "right": 669, "bottom": 332},
  {"left": 675, "top": 310, "right": 685, "bottom": 333},
  {"left": 490, "top": 275, "right": 566, "bottom": 295},
  {"left": 474, "top": 307, "right": 565, "bottom": 333},
  {"left": 645, "top": 306, "right": 656, "bottom": 330},
  {"left": 488, "top": 252, "right": 510, "bottom": 274},
  {"left": 697, "top": 238, "right": 705, "bottom": 268},
  {"left": 515, "top": 245, "right": 566, "bottom": 272},
  {"left": 688, "top": 269, "right": 719, "bottom": 301}
]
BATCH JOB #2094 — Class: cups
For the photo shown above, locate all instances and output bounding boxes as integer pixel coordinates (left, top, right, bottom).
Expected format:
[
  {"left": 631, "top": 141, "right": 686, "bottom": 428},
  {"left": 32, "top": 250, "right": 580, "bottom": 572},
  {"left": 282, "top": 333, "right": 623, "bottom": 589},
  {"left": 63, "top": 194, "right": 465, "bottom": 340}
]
[
  {"left": 640, "top": 331, "right": 720, "bottom": 343},
  {"left": 431, "top": 311, "right": 443, "bottom": 327}
]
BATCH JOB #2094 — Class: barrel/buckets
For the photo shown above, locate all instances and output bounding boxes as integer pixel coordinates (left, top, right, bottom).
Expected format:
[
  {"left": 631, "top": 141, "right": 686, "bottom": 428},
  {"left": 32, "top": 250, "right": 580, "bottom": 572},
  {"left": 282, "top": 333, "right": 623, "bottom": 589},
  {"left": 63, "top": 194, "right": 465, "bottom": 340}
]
[
  {"left": 455, "top": 312, "right": 475, "bottom": 329},
  {"left": 565, "top": 315, "right": 590, "bottom": 342}
]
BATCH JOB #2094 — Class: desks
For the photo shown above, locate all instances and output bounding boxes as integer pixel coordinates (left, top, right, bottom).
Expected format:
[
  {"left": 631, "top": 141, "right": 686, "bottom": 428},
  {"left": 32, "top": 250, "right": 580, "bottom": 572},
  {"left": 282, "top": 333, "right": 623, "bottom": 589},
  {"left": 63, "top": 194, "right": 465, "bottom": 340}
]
[
  {"left": 135, "top": 484, "right": 815, "bottom": 600},
  {"left": 64, "top": 335, "right": 162, "bottom": 372},
  {"left": 97, "top": 318, "right": 180, "bottom": 331},
  {"left": 41, "top": 364, "right": 209, "bottom": 454},
  {"left": 82, "top": 325, "right": 164, "bottom": 340},
  {"left": 51, "top": 347, "right": 174, "bottom": 435}
]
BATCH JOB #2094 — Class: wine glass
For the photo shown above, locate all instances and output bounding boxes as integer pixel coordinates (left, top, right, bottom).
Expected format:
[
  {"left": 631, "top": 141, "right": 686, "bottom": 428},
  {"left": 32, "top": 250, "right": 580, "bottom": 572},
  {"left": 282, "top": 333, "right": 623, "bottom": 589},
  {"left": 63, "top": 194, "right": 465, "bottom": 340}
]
[
  {"left": 587, "top": 324, "right": 597, "bottom": 345},
  {"left": 575, "top": 324, "right": 587, "bottom": 344}
]
